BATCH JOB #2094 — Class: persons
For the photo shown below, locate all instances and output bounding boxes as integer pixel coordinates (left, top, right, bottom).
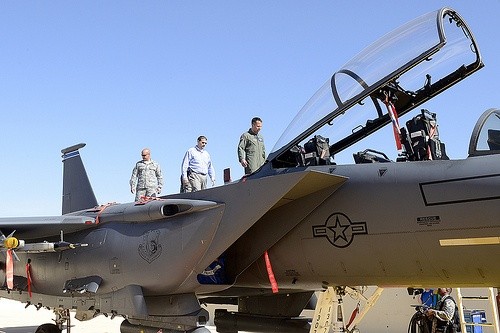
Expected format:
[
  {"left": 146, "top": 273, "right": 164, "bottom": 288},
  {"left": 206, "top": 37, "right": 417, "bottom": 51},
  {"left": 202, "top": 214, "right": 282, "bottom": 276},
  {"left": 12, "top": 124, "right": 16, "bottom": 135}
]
[
  {"left": 420, "top": 288, "right": 458, "bottom": 333},
  {"left": 238, "top": 117, "right": 265, "bottom": 174},
  {"left": 130, "top": 148, "right": 163, "bottom": 202},
  {"left": 182, "top": 136, "right": 216, "bottom": 192}
]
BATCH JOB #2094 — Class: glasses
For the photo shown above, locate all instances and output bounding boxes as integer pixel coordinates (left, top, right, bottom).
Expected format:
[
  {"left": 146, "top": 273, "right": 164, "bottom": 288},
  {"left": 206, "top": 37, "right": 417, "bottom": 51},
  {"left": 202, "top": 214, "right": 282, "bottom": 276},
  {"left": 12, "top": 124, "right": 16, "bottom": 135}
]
[
  {"left": 142, "top": 154, "right": 149, "bottom": 156},
  {"left": 199, "top": 140, "right": 207, "bottom": 144}
]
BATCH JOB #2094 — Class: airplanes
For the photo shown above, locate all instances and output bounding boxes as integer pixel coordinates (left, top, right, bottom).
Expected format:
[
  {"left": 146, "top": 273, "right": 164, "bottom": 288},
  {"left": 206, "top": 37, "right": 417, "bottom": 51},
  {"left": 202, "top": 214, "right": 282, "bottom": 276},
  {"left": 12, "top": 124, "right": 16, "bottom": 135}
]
[{"left": 0, "top": 7, "right": 500, "bottom": 333}]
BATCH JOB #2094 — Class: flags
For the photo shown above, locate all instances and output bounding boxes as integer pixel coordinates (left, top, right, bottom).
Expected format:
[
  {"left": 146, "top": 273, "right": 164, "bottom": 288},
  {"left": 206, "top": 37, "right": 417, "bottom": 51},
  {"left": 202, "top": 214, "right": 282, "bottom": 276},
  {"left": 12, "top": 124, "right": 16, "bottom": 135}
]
[{"left": 387, "top": 100, "right": 401, "bottom": 149}]
